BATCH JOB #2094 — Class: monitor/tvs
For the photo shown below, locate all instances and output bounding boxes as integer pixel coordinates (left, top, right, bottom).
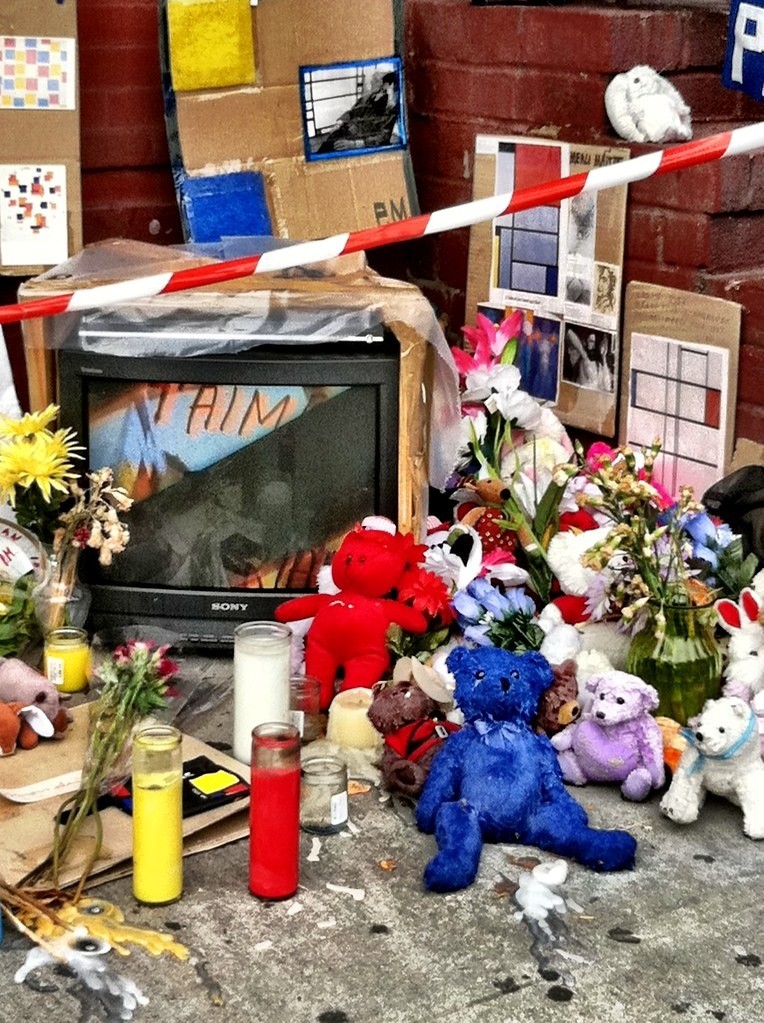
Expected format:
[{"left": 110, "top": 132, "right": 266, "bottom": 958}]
[{"left": 43, "top": 310, "right": 402, "bottom": 661}]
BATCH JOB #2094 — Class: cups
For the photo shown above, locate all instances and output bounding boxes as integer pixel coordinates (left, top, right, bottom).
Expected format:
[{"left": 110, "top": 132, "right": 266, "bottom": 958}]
[
  {"left": 290, "top": 677, "right": 319, "bottom": 740},
  {"left": 132, "top": 725, "right": 183, "bottom": 906},
  {"left": 301, "top": 755, "right": 349, "bottom": 835},
  {"left": 233, "top": 621, "right": 293, "bottom": 765},
  {"left": 45, "top": 627, "right": 89, "bottom": 692},
  {"left": 250, "top": 721, "right": 300, "bottom": 898}
]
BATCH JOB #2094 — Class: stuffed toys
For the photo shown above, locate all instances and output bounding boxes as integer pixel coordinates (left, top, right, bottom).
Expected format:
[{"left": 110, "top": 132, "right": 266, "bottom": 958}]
[
  {"left": 415, "top": 647, "right": 636, "bottom": 891},
  {"left": 534, "top": 649, "right": 664, "bottom": 801},
  {"left": 659, "top": 571, "right": 764, "bottom": 839},
  {"left": 276, "top": 476, "right": 580, "bottom": 794}
]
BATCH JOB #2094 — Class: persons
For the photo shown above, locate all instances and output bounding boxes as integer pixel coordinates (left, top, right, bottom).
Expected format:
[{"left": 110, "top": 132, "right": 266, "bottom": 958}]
[{"left": 564, "top": 324, "right": 615, "bottom": 391}]
